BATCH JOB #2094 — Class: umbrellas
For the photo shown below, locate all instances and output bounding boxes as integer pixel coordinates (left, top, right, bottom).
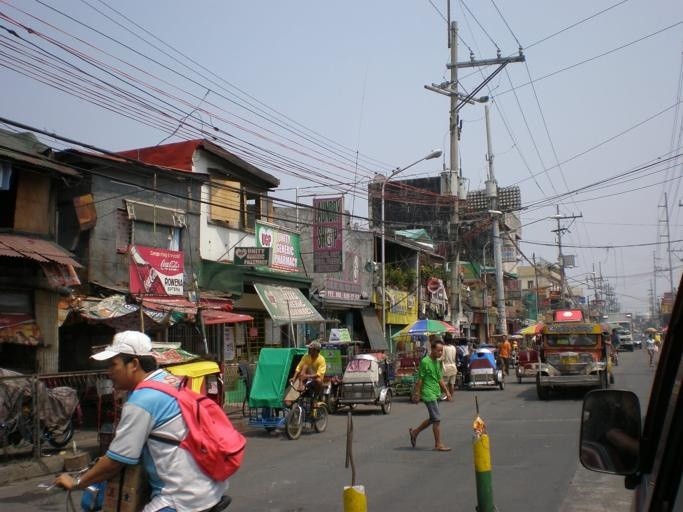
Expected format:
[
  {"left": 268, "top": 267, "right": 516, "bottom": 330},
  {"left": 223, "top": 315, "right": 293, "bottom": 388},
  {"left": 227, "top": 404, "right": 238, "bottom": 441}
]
[
  {"left": 645, "top": 327, "right": 657, "bottom": 333},
  {"left": 391, "top": 317, "right": 456, "bottom": 354},
  {"left": 516, "top": 324, "right": 543, "bottom": 336}
]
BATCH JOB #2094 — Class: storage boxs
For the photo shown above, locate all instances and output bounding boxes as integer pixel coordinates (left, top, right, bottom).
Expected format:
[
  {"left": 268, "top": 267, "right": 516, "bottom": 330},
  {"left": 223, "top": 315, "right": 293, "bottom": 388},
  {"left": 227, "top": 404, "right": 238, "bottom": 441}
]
[
  {"left": 103, "top": 481, "right": 149, "bottom": 512},
  {"left": 107, "top": 461, "right": 146, "bottom": 491}
]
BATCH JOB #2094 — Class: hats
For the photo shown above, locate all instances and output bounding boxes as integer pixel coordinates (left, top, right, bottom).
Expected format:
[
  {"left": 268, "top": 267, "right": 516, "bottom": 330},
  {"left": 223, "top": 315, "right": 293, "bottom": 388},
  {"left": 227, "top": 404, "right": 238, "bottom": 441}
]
[
  {"left": 89, "top": 330, "right": 155, "bottom": 361},
  {"left": 305, "top": 341, "right": 321, "bottom": 350}
]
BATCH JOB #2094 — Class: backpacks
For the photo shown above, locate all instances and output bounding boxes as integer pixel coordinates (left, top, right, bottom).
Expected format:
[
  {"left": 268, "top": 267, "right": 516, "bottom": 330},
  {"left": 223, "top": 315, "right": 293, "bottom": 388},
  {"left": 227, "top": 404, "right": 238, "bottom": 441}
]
[{"left": 134, "top": 374, "right": 247, "bottom": 482}]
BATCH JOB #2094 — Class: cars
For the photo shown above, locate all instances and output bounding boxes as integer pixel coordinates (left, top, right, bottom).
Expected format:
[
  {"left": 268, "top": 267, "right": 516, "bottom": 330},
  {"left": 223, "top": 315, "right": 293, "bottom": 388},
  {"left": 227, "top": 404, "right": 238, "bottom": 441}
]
[
  {"left": 576, "top": 273, "right": 683, "bottom": 511},
  {"left": 633, "top": 337, "right": 642, "bottom": 349}
]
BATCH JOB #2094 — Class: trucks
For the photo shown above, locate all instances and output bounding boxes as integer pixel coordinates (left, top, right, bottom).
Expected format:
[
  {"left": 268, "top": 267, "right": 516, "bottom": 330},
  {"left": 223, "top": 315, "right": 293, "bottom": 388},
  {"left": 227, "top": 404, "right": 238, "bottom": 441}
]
[
  {"left": 613, "top": 328, "right": 632, "bottom": 351},
  {"left": 535, "top": 321, "right": 613, "bottom": 400}
]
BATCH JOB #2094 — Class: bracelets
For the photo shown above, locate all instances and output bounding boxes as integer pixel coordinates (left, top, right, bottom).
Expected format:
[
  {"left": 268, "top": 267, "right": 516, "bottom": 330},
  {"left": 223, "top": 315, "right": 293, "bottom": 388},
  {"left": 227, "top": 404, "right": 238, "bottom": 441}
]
[{"left": 73, "top": 477, "right": 81, "bottom": 489}]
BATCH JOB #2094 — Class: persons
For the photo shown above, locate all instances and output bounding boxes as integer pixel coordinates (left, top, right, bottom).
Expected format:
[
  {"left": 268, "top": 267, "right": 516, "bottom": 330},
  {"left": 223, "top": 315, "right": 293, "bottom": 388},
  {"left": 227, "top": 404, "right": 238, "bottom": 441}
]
[
  {"left": 398, "top": 334, "right": 456, "bottom": 400},
  {"left": 291, "top": 342, "right": 327, "bottom": 409},
  {"left": 498, "top": 335, "right": 511, "bottom": 378},
  {"left": 610, "top": 328, "right": 620, "bottom": 361},
  {"left": 409, "top": 342, "right": 452, "bottom": 452},
  {"left": 57, "top": 331, "right": 230, "bottom": 512},
  {"left": 646, "top": 334, "right": 656, "bottom": 365}
]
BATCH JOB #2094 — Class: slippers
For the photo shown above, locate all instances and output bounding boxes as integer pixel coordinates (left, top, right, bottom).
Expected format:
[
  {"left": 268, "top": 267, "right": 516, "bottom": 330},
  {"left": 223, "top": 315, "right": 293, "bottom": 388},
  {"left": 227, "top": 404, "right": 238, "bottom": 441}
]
[
  {"left": 409, "top": 427, "right": 416, "bottom": 447},
  {"left": 432, "top": 446, "right": 451, "bottom": 451}
]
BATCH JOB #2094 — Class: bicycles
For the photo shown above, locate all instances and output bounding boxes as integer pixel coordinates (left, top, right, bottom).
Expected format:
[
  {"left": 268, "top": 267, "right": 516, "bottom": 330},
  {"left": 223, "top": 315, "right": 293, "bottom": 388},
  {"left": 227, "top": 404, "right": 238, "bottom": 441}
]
[{"left": 37, "top": 458, "right": 231, "bottom": 511}]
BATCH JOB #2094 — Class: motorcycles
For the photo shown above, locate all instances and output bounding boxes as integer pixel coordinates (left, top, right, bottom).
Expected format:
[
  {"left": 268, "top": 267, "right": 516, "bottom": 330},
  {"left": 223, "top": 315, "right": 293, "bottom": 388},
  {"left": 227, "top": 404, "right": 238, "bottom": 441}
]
[
  {"left": 0, "top": 368, "right": 76, "bottom": 452},
  {"left": 96, "top": 360, "right": 230, "bottom": 454}
]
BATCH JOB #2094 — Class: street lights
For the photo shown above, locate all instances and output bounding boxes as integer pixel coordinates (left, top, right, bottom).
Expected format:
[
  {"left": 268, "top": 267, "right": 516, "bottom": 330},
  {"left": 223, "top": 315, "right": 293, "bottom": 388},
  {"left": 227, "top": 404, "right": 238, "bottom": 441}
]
[
  {"left": 461, "top": 207, "right": 503, "bottom": 218},
  {"left": 559, "top": 264, "right": 574, "bottom": 310},
  {"left": 481, "top": 214, "right": 563, "bottom": 335},
  {"left": 532, "top": 260, "right": 559, "bottom": 315},
  {"left": 380, "top": 148, "right": 444, "bottom": 338}
]
